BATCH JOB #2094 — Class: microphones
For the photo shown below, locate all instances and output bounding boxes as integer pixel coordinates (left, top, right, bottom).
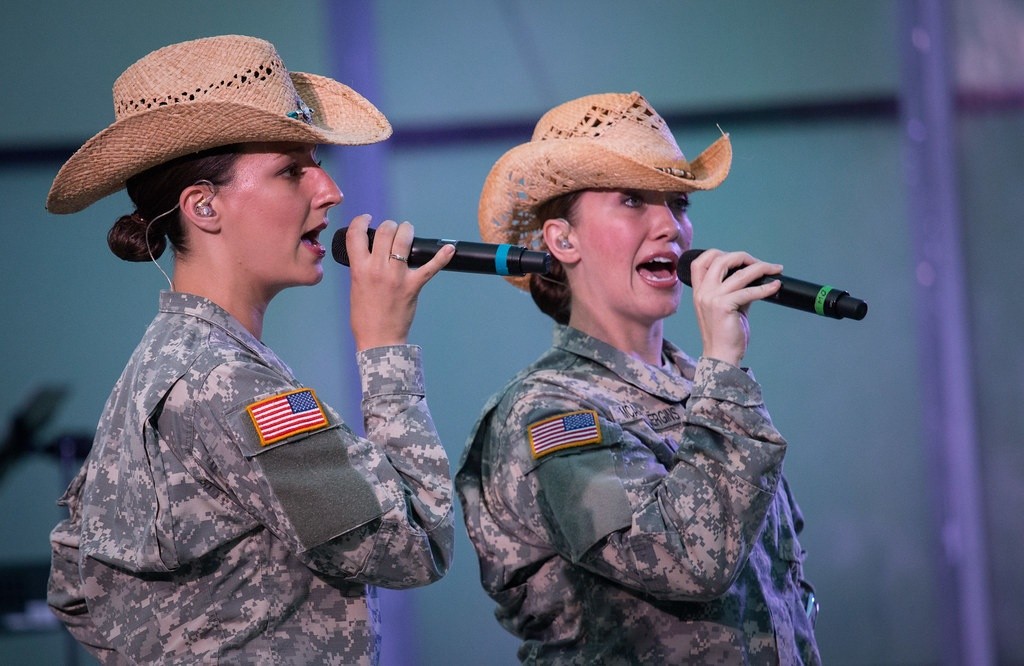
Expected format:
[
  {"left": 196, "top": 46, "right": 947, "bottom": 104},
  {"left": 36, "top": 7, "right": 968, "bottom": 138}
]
[
  {"left": 675, "top": 248, "right": 868, "bottom": 321},
  {"left": 330, "top": 227, "right": 553, "bottom": 276}
]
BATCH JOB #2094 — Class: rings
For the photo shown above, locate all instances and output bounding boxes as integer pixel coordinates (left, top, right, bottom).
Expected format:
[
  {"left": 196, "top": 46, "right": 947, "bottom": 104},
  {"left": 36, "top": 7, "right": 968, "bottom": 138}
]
[{"left": 390, "top": 254, "right": 408, "bottom": 262}]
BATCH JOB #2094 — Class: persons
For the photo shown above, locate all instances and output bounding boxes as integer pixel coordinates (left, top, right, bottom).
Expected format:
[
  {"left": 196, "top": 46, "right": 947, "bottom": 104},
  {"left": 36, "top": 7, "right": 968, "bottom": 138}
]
[
  {"left": 453, "top": 91, "right": 824, "bottom": 666},
  {"left": 47, "top": 35, "right": 456, "bottom": 666}
]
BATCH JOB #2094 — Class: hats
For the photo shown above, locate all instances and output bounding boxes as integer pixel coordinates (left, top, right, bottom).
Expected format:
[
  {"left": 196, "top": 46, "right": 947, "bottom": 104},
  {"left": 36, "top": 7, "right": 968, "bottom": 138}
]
[
  {"left": 477, "top": 90, "right": 734, "bottom": 288},
  {"left": 43, "top": 34, "right": 397, "bottom": 217}
]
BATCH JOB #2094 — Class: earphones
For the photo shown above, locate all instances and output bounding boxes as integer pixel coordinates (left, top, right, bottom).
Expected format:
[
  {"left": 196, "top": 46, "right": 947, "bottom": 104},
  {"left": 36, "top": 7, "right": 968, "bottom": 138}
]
[
  {"left": 194, "top": 197, "right": 215, "bottom": 217},
  {"left": 558, "top": 235, "right": 573, "bottom": 249}
]
